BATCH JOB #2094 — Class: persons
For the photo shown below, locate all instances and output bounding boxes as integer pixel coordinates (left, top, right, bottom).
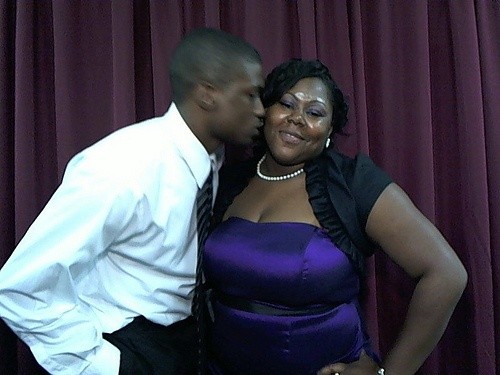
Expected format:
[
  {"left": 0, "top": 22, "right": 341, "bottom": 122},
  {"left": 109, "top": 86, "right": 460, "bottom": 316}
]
[
  {"left": 200, "top": 56, "right": 469, "bottom": 375},
  {"left": 0, "top": 24, "right": 267, "bottom": 374}
]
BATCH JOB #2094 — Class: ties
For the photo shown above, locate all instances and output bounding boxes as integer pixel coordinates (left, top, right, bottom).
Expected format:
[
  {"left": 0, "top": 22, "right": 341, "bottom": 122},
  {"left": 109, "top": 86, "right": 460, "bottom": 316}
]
[{"left": 193, "top": 164, "right": 214, "bottom": 372}]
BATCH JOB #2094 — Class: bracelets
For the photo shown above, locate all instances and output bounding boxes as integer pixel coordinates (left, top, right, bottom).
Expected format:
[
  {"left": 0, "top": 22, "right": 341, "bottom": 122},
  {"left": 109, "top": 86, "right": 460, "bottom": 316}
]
[{"left": 378, "top": 365, "right": 385, "bottom": 375}]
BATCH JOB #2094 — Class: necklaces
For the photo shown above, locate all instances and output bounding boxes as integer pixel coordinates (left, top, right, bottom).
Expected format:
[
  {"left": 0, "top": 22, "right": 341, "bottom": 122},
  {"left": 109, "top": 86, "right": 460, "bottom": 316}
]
[{"left": 256, "top": 150, "right": 304, "bottom": 181}]
